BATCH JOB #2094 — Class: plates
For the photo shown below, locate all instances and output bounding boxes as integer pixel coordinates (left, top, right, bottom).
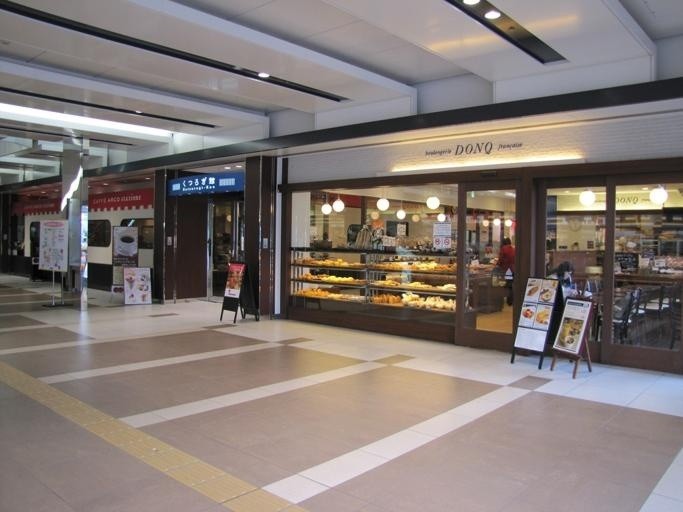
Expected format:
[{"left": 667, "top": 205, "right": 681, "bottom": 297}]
[{"left": 114, "top": 250, "right": 139, "bottom": 258}]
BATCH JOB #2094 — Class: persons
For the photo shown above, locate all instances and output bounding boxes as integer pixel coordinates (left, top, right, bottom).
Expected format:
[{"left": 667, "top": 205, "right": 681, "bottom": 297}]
[
  {"left": 498, "top": 238, "right": 516, "bottom": 306},
  {"left": 544, "top": 260, "right": 593, "bottom": 306}
]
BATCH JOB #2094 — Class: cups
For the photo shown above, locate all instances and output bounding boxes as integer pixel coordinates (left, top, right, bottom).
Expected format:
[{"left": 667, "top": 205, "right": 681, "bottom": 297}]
[
  {"left": 565, "top": 335, "right": 576, "bottom": 350},
  {"left": 117, "top": 233, "right": 136, "bottom": 257}
]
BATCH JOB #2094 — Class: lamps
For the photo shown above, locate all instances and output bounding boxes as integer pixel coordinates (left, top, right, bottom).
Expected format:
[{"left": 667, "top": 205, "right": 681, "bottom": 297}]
[
  {"left": 579, "top": 187, "right": 597, "bottom": 210},
  {"left": 482, "top": 209, "right": 514, "bottom": 228},
  {"left": 377, "top": 184, "right": 448, "bottom": 224},
  {"left": 320, "top": 189, "right": 344, "bottom": 215},
  {"left": 648, "top": 184, "right": 670, "bottom": 206}
]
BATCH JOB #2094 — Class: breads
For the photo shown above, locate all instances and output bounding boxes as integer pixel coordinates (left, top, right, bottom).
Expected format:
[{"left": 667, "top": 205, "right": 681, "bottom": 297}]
[
  {"left": 371, "top": 261, "right": 457, "bottom": 311},
  {"left": 294, "top": 258, "right": 355, "bottom": 300}
]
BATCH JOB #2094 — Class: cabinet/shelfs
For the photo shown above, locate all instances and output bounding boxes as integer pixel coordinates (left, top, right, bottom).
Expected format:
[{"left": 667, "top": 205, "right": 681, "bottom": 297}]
[{"left": 290, "top": 247, "right": 457, "bottom": 312}]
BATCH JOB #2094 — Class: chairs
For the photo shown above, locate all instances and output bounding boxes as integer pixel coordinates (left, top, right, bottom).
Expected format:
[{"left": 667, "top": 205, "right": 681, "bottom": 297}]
[{"left": 571, "top": 274, "right": 683, "bottom": 349}]
